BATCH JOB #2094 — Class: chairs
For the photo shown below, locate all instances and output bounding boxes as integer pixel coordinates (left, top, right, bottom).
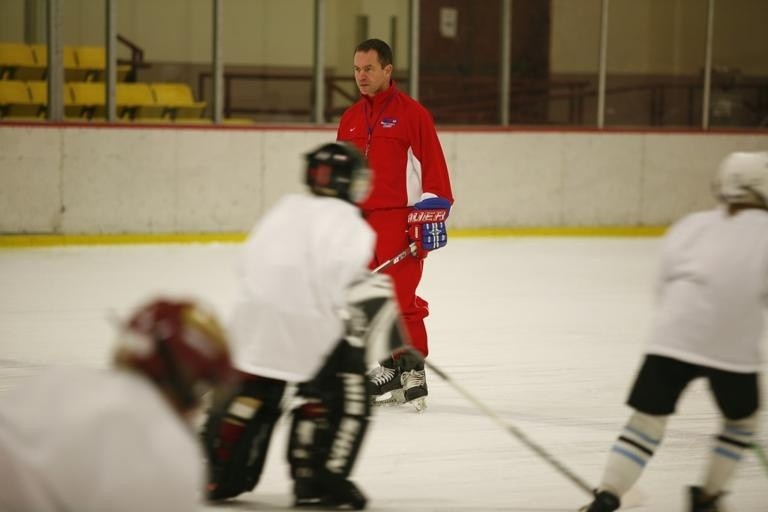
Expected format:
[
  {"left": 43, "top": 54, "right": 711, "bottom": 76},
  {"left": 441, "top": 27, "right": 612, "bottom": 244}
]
[{"left": 2, "top": 40, "right": 210, "bottom": 120}]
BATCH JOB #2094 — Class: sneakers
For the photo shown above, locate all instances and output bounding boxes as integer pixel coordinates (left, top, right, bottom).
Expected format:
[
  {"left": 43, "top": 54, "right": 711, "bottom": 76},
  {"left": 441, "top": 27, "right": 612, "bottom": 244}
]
[
  {"left": 578, "top": 491, "right": 620, "bottom": 512},
  {"left": 295, "top": 478, "right": 366, "bottom": 509},
  {"left": 690, "top": 485, "right": 726, "bottom": 512}
]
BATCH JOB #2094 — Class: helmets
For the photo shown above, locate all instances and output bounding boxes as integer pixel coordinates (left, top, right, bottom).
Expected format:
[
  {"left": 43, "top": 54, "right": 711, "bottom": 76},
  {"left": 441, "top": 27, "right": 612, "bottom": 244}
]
[
  {"left": 716, "top": 152, "right": 767, "bottom": 206},
  {"left": 305, "top": 141, "right": 368, "bottom": 198},
  {"left": 114, "top": 299, "right": 231, "bottom": 413}
]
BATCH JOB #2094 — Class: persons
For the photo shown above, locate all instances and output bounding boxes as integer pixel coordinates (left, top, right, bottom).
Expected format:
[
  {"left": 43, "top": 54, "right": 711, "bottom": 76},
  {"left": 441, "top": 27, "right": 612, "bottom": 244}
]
[
  {"left": 0, "top": 299, "right": 232, "bottom": 512},
  {"left": 334, "top": 38, "right": 455, "bottom": 403},
  {"left": 580, "top": 148, "right": 768, "bottom": 512},
  {"left": 198, "top": 140, "right": 403, "bottom": 512}
]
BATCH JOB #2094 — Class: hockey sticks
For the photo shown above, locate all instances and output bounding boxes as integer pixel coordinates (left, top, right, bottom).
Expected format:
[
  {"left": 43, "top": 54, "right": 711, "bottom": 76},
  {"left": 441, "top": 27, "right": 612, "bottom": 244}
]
[{"left": 406, "top": 347, "right": 643, "bottom": 511}]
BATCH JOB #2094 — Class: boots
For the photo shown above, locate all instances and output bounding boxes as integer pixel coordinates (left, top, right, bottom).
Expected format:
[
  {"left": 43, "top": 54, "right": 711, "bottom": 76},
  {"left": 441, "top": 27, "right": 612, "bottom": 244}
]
[{"left": 366, "top": 354, "right": 428, "bottom": 402}]
